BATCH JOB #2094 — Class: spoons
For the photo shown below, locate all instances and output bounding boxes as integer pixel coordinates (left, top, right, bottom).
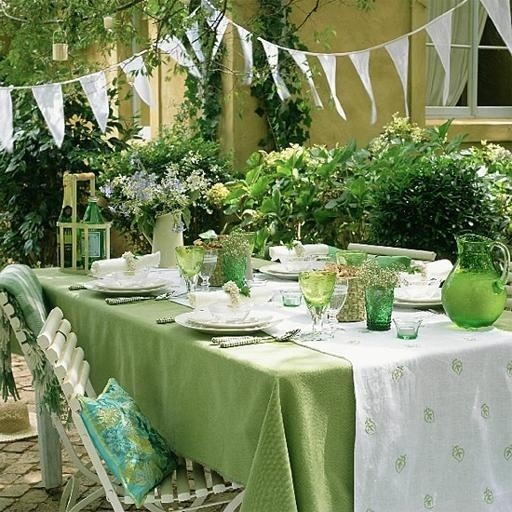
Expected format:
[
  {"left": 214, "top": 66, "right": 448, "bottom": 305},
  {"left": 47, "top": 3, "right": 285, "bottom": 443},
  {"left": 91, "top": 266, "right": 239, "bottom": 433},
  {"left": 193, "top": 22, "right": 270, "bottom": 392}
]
[
  {"left": 210, "top": 327, "right": 301, "bottom": 348},
  {"left": 104, "top": 290, "right": 175, "bottom": 305}
]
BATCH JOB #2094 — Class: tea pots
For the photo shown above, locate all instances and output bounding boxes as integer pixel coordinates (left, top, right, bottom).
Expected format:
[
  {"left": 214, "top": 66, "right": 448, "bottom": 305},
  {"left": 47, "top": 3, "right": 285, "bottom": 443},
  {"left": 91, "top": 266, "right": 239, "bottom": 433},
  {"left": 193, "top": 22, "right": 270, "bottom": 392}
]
[{"left": 441, "top": 231, "right": 511, "bottom": 331}]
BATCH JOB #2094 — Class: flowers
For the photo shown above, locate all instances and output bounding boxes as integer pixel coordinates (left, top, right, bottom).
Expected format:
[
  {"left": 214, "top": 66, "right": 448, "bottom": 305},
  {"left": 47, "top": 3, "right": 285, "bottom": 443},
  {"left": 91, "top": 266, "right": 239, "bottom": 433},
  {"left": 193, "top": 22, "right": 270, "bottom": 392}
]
[{"left": 97, "top": 153, "right": 226, "bottom": 235}]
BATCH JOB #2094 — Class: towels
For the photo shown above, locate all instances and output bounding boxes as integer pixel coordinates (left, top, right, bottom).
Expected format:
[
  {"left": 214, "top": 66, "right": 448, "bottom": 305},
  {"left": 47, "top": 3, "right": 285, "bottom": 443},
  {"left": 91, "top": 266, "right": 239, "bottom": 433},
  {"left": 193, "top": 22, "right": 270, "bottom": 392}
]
[
  {"left": 92, "top": 251, "right": 159, "bottom": 279},
  {"left": 187, "top": 289, "right": 271, "bottom": 309},
  {"left": 269, "top": 245, "right": 328, "bottom": 262},
  {"left": 394, "top": 258, "right": 453, "bottom": 283}
]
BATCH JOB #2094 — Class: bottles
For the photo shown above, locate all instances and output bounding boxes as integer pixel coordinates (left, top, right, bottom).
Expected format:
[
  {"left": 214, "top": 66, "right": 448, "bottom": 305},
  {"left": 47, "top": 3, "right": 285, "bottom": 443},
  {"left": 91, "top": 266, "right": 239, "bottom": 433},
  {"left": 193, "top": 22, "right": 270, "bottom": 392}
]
[
  {"left": 79, "top": 195, "right": 106, "bottom": 270},
  {"left": 56, "top": 173, "right": 82, "bottom": 268}
]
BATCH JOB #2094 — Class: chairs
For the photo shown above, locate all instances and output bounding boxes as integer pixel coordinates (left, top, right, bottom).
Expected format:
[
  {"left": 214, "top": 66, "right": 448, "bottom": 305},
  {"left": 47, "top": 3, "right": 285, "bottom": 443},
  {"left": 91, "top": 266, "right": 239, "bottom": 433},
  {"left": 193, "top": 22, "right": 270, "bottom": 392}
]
[
  {"left": 1, "top": 285, "right": 124, "bottom": 511},
  {"left": 37, "top": 306, "right": 246, "bottom": 512},
  {"left": 347, "top": 243, "right": 436, "bottom": 262}
]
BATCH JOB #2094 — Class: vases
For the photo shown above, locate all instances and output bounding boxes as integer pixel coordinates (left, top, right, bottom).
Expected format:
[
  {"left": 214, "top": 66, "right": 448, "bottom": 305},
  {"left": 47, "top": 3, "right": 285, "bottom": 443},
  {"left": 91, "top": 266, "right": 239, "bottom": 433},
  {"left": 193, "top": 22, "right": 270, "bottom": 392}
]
[{"left": 151, "top": 211, "right": 183, "bottom": 269}]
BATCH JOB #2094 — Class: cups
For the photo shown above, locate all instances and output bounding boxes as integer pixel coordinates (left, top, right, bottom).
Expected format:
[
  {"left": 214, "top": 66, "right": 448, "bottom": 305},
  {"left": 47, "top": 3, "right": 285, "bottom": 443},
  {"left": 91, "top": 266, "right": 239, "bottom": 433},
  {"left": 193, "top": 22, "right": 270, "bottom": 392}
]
[
  {"left": 280, "top": 289, "right": 303, "bottom": 307},
  {"left": 336, "top": 249, "right": 368, "bottom": 268},
  {"left": 392, "top": 317, "right": 423, "bottom": 340},
  {"left": 364, "top": 281, "right": 394, "bottom": 332}
]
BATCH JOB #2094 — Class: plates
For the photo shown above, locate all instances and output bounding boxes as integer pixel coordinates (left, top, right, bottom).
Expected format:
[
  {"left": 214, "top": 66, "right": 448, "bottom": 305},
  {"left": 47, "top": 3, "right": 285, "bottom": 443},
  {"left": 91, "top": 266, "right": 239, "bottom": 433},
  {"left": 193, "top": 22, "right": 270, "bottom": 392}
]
[
  {"left": 258, "top": 262, "right": 321, "bottom": 281},
  {"left": 83, "top": 277, "right": 170, "bottom": 296},
  {"left": 175, "top": 306, "right": 278, "bottom": 335},
  {"left": 392, "top": 284, "right": 443, "bottom": 309}
]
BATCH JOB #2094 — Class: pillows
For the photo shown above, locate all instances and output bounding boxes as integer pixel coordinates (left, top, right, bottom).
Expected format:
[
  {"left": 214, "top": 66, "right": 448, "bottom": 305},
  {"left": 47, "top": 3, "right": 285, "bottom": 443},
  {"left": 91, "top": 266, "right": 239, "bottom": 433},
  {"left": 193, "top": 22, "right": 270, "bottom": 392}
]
[{"left": 78, "top": 378, "right": 177, "bottom": 508}]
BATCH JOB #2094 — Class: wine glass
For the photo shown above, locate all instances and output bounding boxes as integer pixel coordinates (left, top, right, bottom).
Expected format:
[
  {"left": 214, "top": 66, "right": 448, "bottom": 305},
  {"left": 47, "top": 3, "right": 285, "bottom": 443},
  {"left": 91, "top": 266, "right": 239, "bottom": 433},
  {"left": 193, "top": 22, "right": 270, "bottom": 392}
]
[
  {"left": 196, "top": 250, "right": 218, "bottom": 292},
  {"left": 298, "top": 270, "right": 338, "bottom": 341},
  {"left": 322, "top": 278, "right": 349, "bottom": 333},
  {"left": 174, "top": 245, "right": 205, "bottom": 298}
]
